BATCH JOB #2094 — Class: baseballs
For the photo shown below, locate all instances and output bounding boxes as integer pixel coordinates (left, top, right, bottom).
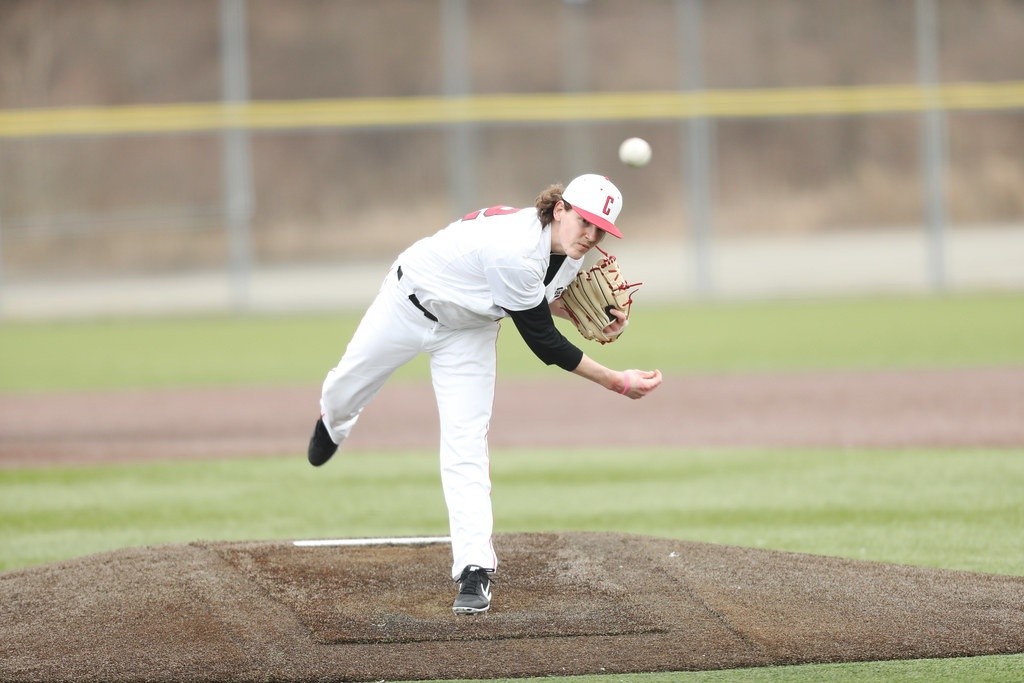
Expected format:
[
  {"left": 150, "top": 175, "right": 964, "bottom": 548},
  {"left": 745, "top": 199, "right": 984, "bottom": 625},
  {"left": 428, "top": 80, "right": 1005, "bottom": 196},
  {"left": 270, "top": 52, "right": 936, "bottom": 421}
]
[{"left": 618, "top": 136, "right": 653, "bottom": 169}]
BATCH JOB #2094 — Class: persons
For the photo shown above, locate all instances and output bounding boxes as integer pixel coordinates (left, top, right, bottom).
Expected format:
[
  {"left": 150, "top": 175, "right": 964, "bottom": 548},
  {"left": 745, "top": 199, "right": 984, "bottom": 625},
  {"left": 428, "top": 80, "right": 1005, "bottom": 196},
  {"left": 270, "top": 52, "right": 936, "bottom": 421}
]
[{"left": 307, "top": 170, "right": 662, "bottom": 615}]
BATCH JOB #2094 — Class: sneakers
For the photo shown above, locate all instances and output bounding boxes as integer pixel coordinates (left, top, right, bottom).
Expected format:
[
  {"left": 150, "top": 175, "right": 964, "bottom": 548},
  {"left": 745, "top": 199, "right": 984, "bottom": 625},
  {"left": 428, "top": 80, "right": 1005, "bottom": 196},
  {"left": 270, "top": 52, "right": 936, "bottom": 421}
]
[
  {"left": 308, "top": 413, "right": 339, "bottom": 466},
  {"left": 451, "top": 565, "right": 495, "bottom": 615}
]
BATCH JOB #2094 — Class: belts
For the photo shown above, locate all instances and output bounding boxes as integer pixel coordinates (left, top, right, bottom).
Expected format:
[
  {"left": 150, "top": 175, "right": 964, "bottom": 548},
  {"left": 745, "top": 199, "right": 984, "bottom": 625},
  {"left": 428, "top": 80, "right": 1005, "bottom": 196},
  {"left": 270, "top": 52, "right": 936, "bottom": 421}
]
[{"left": 397, "top": 266, "right": 438, "bottom": 322}]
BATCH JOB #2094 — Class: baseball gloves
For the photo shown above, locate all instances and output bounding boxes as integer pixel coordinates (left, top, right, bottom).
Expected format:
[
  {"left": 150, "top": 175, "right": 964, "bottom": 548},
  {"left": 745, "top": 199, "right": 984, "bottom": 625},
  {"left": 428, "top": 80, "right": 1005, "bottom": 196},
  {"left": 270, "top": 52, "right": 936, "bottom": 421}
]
[{"left": 557, "top": 256, "right": 631, "bottom": 346}]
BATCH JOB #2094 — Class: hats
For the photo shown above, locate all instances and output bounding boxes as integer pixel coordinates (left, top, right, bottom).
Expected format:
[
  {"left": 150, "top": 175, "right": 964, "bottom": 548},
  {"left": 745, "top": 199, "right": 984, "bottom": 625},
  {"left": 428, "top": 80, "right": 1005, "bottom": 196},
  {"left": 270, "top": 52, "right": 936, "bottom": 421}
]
[{"left": 562, "top": 174, "right": 623, "bottom": 240}]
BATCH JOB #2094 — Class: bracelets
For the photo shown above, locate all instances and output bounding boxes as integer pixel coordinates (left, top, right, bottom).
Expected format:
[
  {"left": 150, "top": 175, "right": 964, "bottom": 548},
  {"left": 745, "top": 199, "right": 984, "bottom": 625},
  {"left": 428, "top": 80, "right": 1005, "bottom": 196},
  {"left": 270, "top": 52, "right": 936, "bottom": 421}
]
[{"left": 619, "top": 371, "right": 631, "bottom": 395}]
